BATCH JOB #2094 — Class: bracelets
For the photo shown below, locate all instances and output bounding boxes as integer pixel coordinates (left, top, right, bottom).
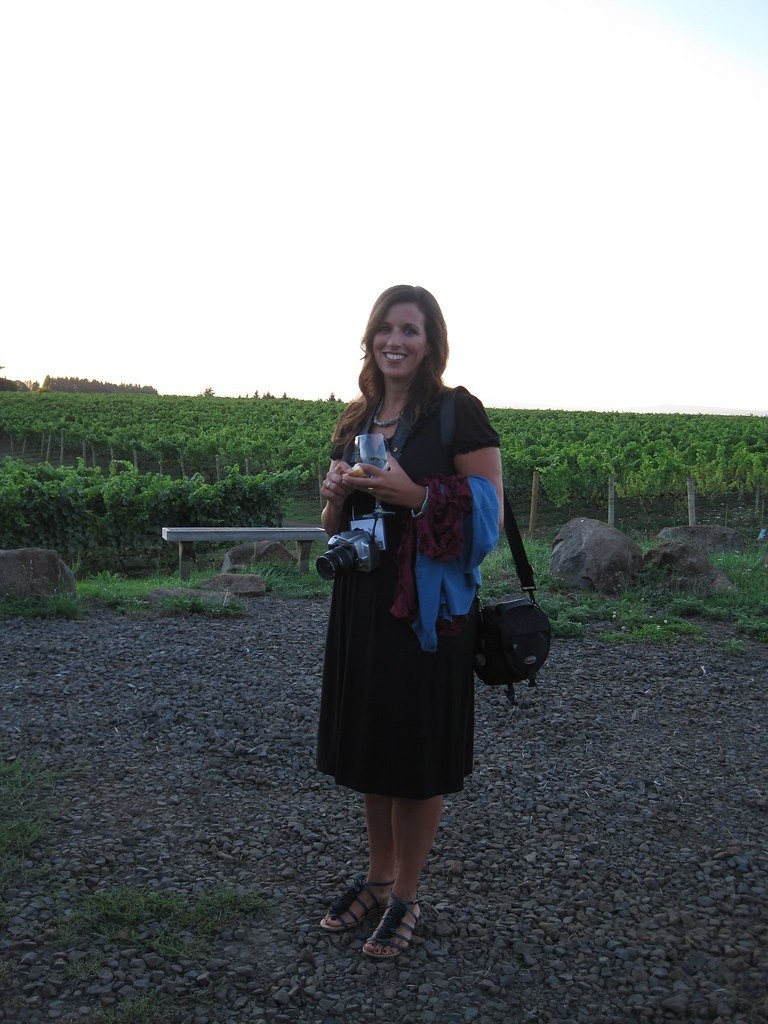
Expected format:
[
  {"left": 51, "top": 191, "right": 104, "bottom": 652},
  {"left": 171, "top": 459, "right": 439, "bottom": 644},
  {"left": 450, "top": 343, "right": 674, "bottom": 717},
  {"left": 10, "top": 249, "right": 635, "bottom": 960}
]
[{"left": 411, "top": 486, "right": 430, "bottom": 518}]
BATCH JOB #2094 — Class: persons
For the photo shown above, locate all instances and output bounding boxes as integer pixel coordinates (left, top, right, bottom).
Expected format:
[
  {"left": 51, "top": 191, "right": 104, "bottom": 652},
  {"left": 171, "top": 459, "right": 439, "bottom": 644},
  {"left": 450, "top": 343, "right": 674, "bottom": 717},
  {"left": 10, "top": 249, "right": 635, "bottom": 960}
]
[{"left": 317, "top": 282, "right": 504, "bottom": 960}]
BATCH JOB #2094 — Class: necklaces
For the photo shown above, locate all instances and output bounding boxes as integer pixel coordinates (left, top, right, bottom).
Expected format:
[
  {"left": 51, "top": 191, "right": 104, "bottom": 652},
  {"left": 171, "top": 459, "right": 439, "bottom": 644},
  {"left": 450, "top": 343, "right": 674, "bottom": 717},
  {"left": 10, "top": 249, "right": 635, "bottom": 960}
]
[{"left": 373, "top": 397, "right": 404, "bottom": 427}]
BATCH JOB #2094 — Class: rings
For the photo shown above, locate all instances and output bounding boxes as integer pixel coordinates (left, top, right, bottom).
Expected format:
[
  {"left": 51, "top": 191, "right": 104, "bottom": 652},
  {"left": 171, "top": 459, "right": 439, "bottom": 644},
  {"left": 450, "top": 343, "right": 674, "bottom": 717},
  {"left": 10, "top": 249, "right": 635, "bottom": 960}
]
[{"left": 326, "top": 480, "right": 332, "bottom": 488}]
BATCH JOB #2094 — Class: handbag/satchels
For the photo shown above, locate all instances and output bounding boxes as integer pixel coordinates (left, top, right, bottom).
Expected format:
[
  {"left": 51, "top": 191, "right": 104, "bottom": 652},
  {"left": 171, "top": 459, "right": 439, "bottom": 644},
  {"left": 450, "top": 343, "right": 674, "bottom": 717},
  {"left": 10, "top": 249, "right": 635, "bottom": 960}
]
[{"left": 473, "top": 597, "right": 551, "bottom": 686}]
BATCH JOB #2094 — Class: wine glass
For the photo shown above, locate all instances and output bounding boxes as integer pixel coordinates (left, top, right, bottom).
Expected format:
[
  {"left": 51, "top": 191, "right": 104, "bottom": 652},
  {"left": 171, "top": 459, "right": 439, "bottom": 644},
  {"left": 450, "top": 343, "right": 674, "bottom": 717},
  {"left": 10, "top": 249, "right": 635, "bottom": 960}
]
[{"left": 354, "top": 433, "right": 397, "bottom": 519}]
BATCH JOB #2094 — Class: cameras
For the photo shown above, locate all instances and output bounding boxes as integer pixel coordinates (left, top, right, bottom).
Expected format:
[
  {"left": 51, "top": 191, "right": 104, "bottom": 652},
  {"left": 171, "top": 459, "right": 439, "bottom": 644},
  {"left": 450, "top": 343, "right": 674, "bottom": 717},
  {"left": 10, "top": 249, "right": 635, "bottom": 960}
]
[{"left": 315, "top": 527, "right": 382, "bottom": 582}]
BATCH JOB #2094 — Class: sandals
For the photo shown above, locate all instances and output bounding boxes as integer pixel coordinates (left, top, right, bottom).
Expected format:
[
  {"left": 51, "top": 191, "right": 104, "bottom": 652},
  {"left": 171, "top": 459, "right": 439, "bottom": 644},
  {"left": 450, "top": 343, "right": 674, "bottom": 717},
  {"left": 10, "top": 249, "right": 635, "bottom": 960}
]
[
  {"left": 362, "top": 891, "right": 421, "bottom": 959},
  {"left": 320, "top": 874, "right": 396, "bottom": 931}
]
